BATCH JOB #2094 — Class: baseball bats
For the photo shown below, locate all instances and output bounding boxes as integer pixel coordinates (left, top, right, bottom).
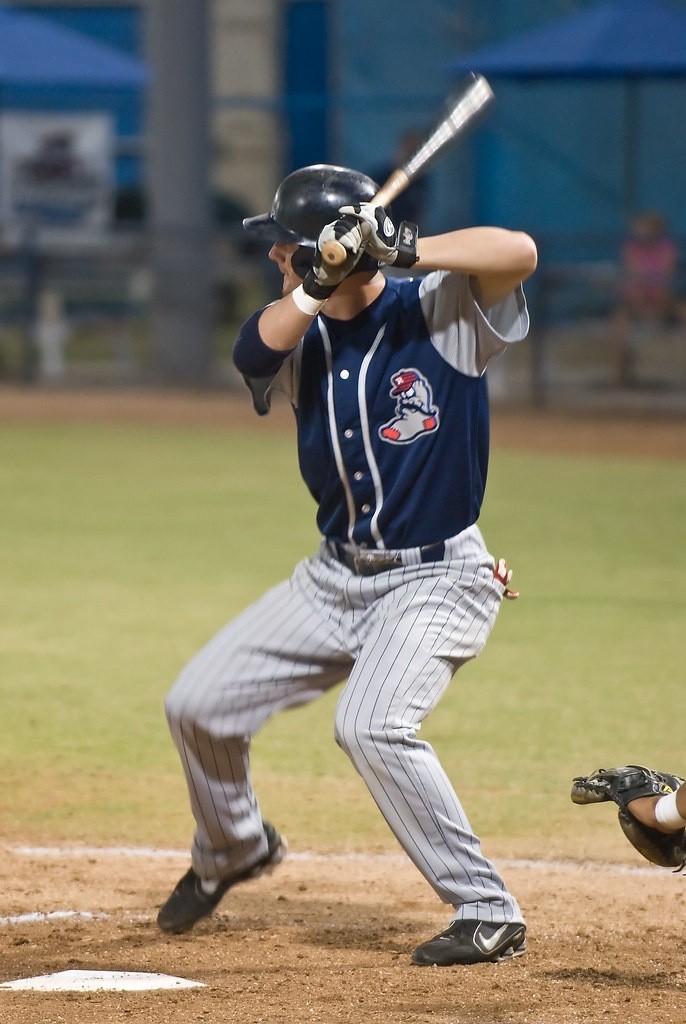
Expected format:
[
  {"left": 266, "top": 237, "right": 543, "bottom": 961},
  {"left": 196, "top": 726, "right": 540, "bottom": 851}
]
[{"left": 321, "top": 76, "right": 497, "bottom": 262}]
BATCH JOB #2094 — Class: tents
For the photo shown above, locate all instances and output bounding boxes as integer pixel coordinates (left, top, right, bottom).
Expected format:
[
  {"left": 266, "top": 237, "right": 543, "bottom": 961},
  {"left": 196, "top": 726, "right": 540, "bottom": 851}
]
[{"left": 448, "top": 0, "right": 686, "bottom": 262}]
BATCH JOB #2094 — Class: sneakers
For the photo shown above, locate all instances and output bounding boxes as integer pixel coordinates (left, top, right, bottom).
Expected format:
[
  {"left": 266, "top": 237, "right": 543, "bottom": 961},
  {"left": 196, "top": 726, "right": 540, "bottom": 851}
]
[
  {"left": 410, "top": 919, "right": 526, "bottom": 966},
  {"left": 156, "top": 821, "right": 288, "bottom": 935}
]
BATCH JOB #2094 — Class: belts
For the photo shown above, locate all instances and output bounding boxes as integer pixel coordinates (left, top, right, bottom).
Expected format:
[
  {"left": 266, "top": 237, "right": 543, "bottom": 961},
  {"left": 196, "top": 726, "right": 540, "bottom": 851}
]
[{"left": 324, "top": 537, "right": 446, "bottom": 577}]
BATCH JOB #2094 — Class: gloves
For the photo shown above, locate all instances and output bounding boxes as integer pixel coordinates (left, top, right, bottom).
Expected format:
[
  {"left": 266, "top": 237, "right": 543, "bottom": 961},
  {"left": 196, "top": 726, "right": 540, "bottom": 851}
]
[
  {"left": 302, "top": 213, "right": 371, "bottom": 300},
  {"left": 338, "top": 202, "right": 420, "bottom": 269},
  {"left": 496, "top": 558, "right": 520, "bottom": 602}
]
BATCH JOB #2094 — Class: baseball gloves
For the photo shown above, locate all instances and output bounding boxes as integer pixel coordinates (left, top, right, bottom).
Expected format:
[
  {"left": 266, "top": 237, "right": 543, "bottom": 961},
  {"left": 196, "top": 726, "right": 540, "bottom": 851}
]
[{"left": 571, "top": 765, "right": 686, "bottom": 872}]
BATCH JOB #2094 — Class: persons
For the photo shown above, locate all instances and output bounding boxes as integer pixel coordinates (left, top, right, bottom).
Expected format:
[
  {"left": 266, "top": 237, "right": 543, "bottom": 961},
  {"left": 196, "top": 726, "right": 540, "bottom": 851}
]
[
  {"left": 571, "top": 764, "right": 686, "bottom": 879},
  {"left": 15, "top": 127, "right": 430, "bottom": 377},
  {"left": 156, "top": 166, "right": 537, "bottom": 965},
  {"left": 615, "top": 216, "right": 685, "bottom": 331}
]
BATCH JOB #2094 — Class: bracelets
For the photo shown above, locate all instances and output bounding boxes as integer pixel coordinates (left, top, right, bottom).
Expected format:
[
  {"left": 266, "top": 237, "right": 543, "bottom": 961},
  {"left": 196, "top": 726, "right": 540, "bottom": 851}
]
[
  {"left": 655, "top": 790, "right": 686, "bottom": 830},
  {"left": 292, "top": 283, "right": 329, "bottom": 315}
]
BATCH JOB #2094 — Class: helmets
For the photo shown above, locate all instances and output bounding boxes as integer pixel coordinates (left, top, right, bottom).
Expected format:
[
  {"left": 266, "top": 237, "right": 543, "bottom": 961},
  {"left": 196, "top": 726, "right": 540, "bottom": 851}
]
[{"left": 242, "top": 165, "right": 392, "bottom": 282}]
